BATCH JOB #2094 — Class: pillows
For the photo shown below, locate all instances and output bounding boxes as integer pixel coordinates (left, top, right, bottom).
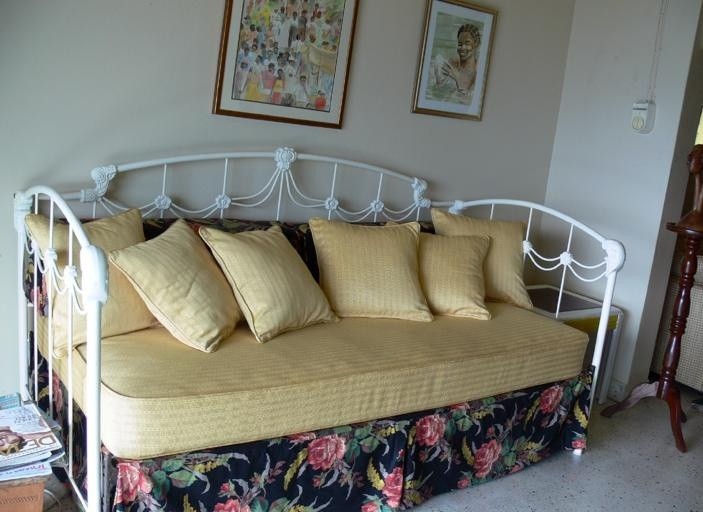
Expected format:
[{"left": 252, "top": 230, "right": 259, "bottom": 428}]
[
  {"left": 106, "top": 216, "right": 243, "bottom": 353},
  {"left": 308, "top": 214, "right": 433, "bottom": 323},
  {"left": 25, "top": 204, "right": 161, "bottom": 360},
  {"left": 386, "top": 219, "right": 494, "bottom": 322},
  {"left": 197, "top": 222, "right": 340, "bottom": 344},
  {"left": 430, "top": 205, "right": 536, "bottom": 313}
]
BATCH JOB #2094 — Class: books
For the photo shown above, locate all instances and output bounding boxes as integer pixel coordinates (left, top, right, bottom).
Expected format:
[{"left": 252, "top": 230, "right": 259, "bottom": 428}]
[{"left": 1, "top": 385, "right": 65, "bottom": 482}]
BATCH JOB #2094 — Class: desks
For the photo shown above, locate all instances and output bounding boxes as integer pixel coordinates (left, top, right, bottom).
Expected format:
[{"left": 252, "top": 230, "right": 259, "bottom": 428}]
[
  {"left": 520, "top": 280, "right": 624, "bottom": 406},
  {"left": 0, "top": 459, "right": 53, "bottom": 511}
]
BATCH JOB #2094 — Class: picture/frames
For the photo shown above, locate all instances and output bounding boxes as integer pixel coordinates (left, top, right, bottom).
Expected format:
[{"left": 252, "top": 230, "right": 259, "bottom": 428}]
[
  {"left": 408, "top": 1, "right": 499, "bottom": 120},
  {"left": 210, "top": 0, "right": 361, "bottom": 130}
]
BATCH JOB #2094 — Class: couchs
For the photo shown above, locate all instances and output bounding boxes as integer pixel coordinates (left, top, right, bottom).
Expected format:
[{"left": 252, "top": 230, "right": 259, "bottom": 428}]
[{"left": 14, "top": 145, "right": 625, "bottom": 510}]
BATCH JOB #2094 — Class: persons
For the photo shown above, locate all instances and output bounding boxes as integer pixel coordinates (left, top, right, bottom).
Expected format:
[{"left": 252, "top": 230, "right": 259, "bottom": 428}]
[
  {"left": 441, "top": 22, "right": 482, "bottom": 93},
  {"left": 236, "top": 0, "right": 342, "bottom": 111}
]
